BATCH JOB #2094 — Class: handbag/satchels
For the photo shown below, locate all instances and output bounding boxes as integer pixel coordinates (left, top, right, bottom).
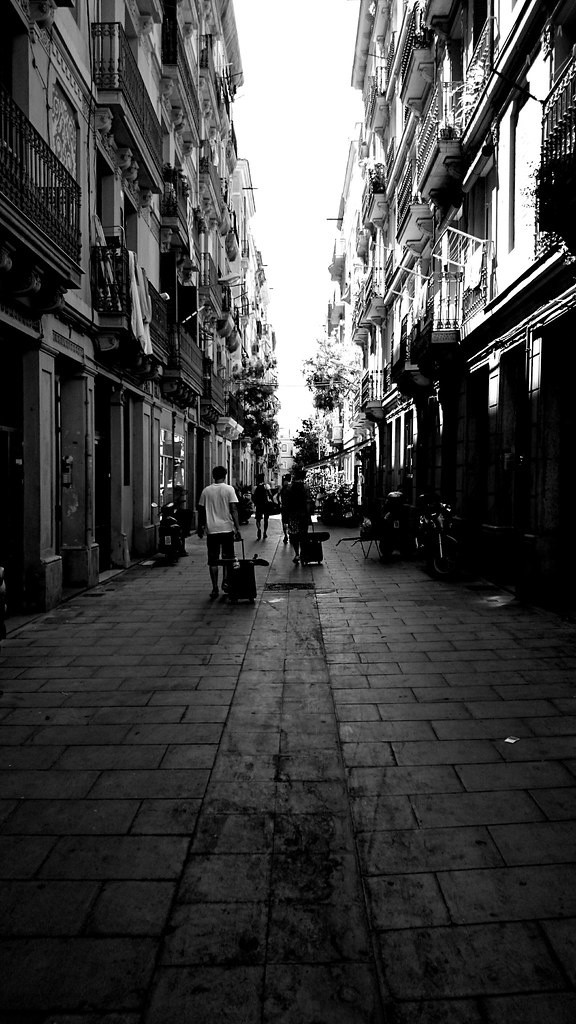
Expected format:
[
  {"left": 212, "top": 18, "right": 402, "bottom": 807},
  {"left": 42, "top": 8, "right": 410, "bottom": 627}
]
[{"left": 267, "top": 501, "right": 280, "bottom": 515}]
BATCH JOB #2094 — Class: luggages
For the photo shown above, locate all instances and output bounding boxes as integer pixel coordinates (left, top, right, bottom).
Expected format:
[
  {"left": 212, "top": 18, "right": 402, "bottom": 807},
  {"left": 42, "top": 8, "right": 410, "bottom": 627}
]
[
  {"left": 299, "top": 523, "right": 323, "bottom": 566},
  {"left": 221, "top": 538, "right": 260, "bottom": 604}
]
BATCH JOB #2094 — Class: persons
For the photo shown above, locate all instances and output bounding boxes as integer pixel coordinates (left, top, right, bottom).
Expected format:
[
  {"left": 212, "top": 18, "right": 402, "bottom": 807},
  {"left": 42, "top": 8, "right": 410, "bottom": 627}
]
[
  {"left": 198, "top": 466, "right": 242, "bottom": 597},
  {"left": 250, "top": 471, "right": 318, "bottom": 563}
]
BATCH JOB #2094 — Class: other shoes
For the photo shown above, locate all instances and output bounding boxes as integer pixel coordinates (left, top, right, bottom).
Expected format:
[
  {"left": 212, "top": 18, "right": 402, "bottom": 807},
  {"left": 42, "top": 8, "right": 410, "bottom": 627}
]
[
  {"left": 210, "top": 585, "right": 219, "bottom": 595},
  {"left": 262, "top": 534, "right": 268, "bottom": 538},
  {"left": 283, "top": 537, "right": 288, "bottom": 542},
  {"left": 257, "top": 530, "right": 261, "bottom": 538},
  {"left": 292, "top": 555, "right": 300, "bottom": 564}
]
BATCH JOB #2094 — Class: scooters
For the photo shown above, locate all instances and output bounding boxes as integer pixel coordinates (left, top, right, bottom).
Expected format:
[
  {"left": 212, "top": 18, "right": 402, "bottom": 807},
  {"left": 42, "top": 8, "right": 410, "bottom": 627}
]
[
  {"left": 416, "top": 493, "right": 463, "bottom": 579},
  {"left": 150, "top": 490, "right": 189, "bottom": 567}
]
